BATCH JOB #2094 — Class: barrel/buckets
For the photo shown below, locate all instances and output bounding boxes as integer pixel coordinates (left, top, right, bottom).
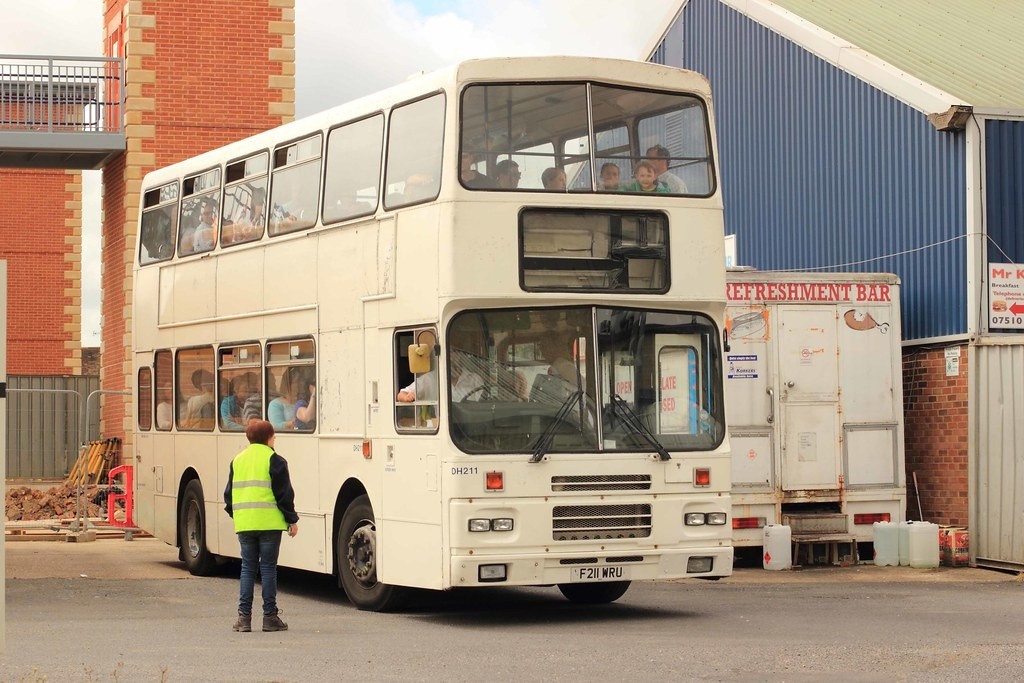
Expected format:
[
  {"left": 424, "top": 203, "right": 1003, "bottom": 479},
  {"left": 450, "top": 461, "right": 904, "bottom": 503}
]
[
  {"left": 873, "top": 521, "right": 940, "bottom": 569},
  {"left": 763, "top": 524, "right": 792, "bottom": 570}
]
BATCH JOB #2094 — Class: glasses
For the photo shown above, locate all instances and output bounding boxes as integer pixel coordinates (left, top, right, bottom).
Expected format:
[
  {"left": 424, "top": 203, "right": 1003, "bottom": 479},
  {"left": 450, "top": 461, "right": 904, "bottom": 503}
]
[
  {"left": 203, "top": 211, "right": 215, "bottom": 216},
  {"left": 504, "top": 171, "right": 521, "bottom": 178}
]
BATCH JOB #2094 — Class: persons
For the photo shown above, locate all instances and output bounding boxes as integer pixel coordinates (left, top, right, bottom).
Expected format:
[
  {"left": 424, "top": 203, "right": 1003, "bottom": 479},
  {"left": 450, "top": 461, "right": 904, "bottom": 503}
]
[
  {"left": 156, "top": 144, "right": 691, "bottom": 432},
  {"left": 223, "top": 417, "right": 300, "bottom": 632}
]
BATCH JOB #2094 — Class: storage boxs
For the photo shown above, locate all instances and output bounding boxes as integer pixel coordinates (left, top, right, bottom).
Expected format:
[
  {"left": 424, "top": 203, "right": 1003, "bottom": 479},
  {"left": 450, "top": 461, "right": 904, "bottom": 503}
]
[{"left": 939, "top": 524, "right": 970, "bottom": 567}]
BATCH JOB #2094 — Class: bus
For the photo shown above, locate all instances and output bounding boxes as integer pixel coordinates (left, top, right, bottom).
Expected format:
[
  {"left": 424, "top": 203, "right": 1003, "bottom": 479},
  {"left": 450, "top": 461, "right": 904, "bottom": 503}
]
[{"left": 128, "top": 57, "right": 736, "bottom": 613}]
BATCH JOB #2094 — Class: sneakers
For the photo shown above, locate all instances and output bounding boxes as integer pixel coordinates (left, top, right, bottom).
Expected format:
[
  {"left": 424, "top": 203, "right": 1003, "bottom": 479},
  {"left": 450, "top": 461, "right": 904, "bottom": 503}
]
[
  {"left": 262, "top": 609, "right": 288, "bottom": 632},
  {"left": 232, "top": 610, "right": 252, "bottom": 632}
]
